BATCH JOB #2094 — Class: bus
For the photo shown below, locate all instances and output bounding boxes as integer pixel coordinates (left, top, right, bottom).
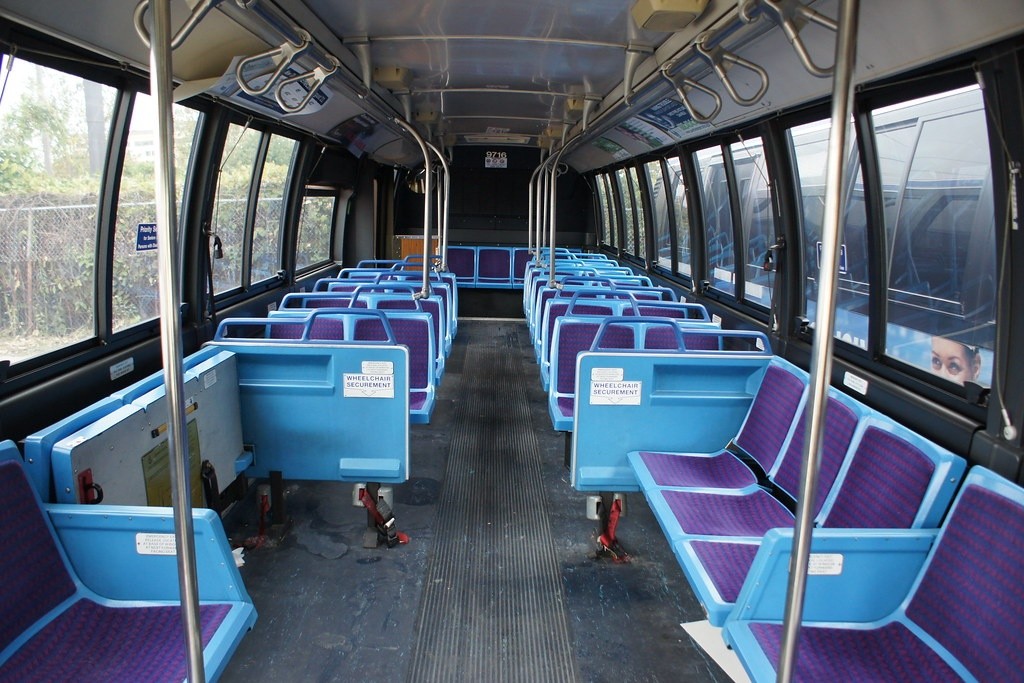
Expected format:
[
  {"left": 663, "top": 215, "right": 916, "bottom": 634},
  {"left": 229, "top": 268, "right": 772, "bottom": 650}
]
[{"left": 640, "top": 82, "right": 997, "bottom": 393}]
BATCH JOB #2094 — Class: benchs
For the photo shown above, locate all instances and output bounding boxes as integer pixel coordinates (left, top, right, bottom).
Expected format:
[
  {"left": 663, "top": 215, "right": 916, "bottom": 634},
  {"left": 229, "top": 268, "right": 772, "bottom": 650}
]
[{"left": 1, "top": 240, "right": 1023, "bottom": 683}]
